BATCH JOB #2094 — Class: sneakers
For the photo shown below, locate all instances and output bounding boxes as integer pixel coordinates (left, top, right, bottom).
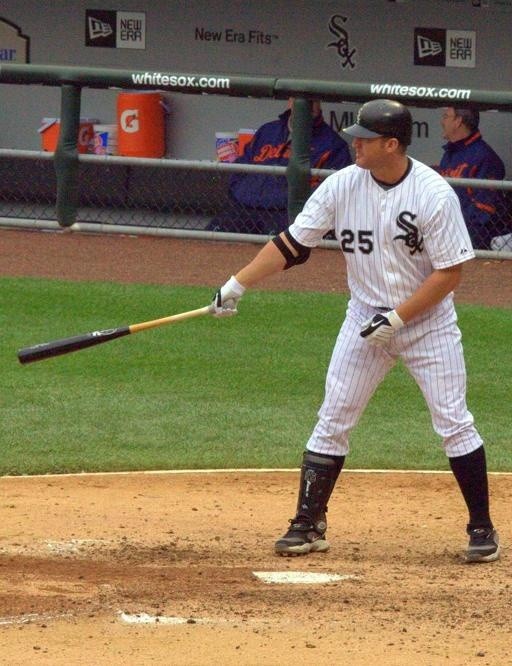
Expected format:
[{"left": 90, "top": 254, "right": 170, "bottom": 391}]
[
  {"left": 464, "top": 524, "right": 500, "bottom": 563},
  {"left": 275, "top": 510, "right": 330, "bottom": 557}
]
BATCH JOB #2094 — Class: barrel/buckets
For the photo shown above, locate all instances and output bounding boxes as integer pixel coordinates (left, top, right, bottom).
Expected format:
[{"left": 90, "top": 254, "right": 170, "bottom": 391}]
[
  {"left": 37, "top": 117, "right": 98, "bottom": 155},
  {"left": 215, "top": 130, "right": 237, "bottom": 163},
  {"left": 93, "top": 123, "right": 117, "bottom": 154},
  {"left": 117, "top": 89, "right": 170, "bottom": 158},
  {"left": 238, "top": 127, "right": 256, "bottom": 157}
]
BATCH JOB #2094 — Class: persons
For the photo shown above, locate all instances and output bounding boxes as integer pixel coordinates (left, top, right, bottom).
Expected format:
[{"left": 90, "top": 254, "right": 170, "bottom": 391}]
[
  {"left": 426, "top": 105, "right": 511, "bottom": 250},
  {"left": 201, "top": 94, "right": 354, "bottom": 242},
  {"left": 207, "top": 96, "right": 501, "bottom": 565}
]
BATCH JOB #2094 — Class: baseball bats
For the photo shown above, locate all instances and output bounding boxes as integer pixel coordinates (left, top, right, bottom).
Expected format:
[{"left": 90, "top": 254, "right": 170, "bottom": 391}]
[{"left": 18, "top": 300, "right": 235, "bottom": 364}]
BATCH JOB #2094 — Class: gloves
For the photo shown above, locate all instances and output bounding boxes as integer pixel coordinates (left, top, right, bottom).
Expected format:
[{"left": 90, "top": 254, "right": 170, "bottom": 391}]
[
  {"left": 212, "top": 275, "right": 245, "bottom": 315},
  {"left": 360, "top": 309, "right": 404, "bottom": 347}
]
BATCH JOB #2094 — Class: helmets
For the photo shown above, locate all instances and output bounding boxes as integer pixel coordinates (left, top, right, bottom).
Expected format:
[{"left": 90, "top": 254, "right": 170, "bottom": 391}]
[{"left": 343, "top": 99, "right": 413, "bottom": 139}]
